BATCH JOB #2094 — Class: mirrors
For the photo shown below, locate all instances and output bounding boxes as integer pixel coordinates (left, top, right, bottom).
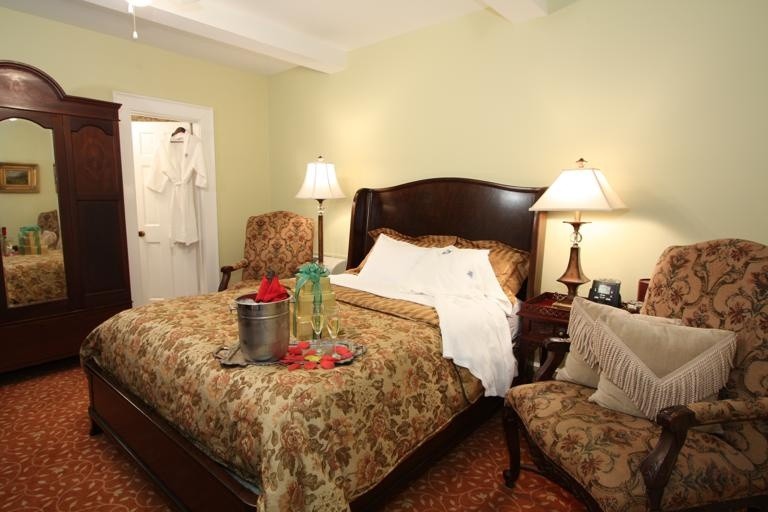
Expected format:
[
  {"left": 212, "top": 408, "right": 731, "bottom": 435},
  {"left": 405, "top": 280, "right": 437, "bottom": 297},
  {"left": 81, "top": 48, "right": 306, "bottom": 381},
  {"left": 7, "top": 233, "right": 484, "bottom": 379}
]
[{"left": 0, "top": 109, "right": 72, "bottom": 308}]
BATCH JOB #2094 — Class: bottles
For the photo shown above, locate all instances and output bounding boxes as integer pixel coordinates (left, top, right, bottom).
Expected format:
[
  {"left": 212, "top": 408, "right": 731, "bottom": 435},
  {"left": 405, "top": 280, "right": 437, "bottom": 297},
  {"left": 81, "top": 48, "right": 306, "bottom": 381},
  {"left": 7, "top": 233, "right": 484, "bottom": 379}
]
[
  {"left": 1, "top": 227, "right": 12, "bottom": 256},
  {"left": 264, "top": 269, "right": 275, "bottom": 284}
]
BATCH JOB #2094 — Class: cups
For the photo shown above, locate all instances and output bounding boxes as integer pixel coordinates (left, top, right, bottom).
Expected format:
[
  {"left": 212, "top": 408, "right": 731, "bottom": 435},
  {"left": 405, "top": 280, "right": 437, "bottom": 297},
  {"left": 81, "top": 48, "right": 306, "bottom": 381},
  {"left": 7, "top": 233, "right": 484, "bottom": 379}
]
[{"left": 638, "top": 278, "right": 651, "bottom": 303}]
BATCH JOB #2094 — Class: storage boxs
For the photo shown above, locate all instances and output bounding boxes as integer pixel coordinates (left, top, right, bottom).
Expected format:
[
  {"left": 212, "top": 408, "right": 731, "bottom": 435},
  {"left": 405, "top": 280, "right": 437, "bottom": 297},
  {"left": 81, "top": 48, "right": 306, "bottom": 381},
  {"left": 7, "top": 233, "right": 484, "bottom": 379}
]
[
  {"left": 288, "top": 287, "right": 337, "bottom": 317},
  {"left": 285, "top": 306, "right": 342, "bottom": 342},
  {"left": 294, "top": 272, "right": 331, "bottom": 294}
]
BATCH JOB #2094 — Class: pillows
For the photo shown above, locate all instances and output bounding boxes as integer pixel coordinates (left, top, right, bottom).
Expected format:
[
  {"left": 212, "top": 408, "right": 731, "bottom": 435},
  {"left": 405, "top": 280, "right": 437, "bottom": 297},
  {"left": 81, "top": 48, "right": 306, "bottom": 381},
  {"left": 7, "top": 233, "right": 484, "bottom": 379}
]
[
  {"left": 552, "top": 296, "right": 681, "bottom": 389},
  {"left": 587, "top": 305, "right": 739, "bottom": 423},
  {"left": 453, "top": 238, "right": 531, "bottom": 311},
  {"left": 353, "top": 225, "right": 457, "bottom": 288}
]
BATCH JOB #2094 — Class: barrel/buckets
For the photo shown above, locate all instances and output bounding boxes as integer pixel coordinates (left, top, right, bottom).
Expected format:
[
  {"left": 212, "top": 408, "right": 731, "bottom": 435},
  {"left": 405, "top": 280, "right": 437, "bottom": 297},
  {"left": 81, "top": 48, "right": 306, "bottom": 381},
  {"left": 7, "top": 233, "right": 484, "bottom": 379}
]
[{"left": 228, "top": 290, "right": 293, "bottom": 364}]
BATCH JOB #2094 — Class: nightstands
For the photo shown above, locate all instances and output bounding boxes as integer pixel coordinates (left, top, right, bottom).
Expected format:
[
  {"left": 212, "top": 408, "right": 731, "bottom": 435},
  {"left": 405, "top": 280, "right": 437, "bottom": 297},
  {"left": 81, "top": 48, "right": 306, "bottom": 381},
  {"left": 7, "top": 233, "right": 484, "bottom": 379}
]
[{"left": 513, "top": 291, "right": 640, "bottom": 387}]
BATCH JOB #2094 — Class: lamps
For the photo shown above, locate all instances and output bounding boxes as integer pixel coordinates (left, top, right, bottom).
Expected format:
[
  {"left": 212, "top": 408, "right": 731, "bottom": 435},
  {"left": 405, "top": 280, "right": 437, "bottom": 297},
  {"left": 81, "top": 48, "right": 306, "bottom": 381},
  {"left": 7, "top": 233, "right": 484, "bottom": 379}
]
[
  {"left": 528, "top": 157, "right": 629, "bottom": 310},
  {"left": 292, "top": 154, "right": 348, "bottom": 267}
]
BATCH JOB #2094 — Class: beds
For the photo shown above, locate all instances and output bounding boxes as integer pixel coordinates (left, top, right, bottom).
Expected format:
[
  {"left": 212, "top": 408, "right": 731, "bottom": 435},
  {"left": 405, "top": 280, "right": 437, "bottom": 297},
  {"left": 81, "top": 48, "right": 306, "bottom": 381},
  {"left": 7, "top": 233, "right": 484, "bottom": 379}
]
[
  {"left": 78, "top": 176, "right": 553, "bottom": 509},
  {"left": 3, "top": 244, "right": 67, "bottom": 306}
]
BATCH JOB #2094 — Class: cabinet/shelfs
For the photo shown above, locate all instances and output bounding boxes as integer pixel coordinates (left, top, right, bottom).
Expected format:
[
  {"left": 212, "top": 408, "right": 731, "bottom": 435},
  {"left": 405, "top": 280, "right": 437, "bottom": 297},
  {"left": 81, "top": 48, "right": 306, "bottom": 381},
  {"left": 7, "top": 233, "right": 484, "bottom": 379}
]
[{"left": 2, "top": 57, "right": 137, "bottom": 388}]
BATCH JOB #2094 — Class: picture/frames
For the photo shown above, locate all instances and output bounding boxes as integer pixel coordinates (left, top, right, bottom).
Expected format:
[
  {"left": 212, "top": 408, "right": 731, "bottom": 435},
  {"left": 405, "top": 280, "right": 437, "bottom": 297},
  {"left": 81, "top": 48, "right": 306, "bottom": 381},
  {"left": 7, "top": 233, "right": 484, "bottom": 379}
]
[{"left": 0, "top": 161, "right": 42, "bottom": 197}]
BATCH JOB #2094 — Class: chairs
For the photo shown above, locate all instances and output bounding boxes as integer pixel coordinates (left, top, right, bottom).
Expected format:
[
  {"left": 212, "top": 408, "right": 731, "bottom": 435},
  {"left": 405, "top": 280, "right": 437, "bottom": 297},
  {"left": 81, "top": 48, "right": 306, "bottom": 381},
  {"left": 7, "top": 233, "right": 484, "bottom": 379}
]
[
  {"left": 32, "top": 210, "right": 59, "bottom": 250},
  {"left": 216, "top": 210, "right": 315, "bottom": 297},
  {"left": 497, "top": 236, "right": 766, "bottom": 508}
]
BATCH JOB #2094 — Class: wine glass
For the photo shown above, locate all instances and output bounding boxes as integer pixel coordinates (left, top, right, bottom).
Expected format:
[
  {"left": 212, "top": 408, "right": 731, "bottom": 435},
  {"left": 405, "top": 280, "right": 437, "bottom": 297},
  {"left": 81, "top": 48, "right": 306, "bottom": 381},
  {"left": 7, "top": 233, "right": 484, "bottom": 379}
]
[
  {"left": 307, "top": 306, "right": 325, "bottom": 354},
  {"left": 324, "top": 304, "right": 344, "bottom": 360}
]
